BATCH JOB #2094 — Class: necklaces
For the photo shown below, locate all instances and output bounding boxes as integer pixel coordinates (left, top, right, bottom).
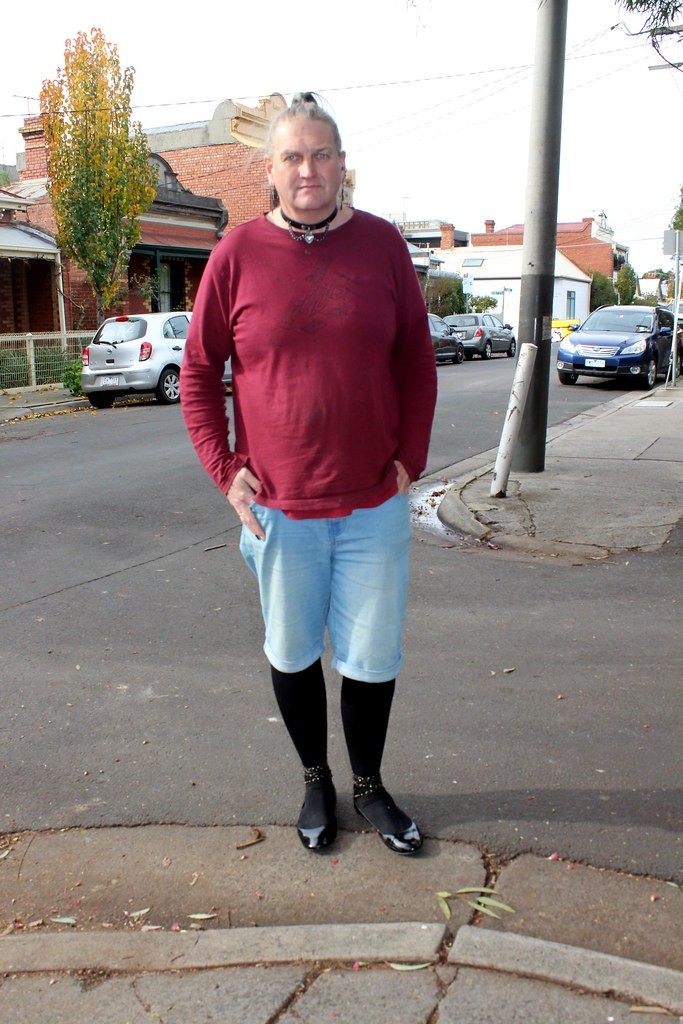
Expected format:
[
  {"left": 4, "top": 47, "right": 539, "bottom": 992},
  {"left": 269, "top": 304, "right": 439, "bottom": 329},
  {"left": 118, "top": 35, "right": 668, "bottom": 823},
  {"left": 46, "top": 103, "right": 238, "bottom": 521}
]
[{"left": 280, "top": 207, "right": 338, "bottom": 244}]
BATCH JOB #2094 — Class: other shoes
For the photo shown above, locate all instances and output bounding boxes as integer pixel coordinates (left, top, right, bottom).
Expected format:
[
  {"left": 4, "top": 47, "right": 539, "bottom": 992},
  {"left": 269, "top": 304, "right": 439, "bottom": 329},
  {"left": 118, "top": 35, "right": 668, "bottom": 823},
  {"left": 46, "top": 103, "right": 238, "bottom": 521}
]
[
  {"left": 297, "top": 783, "right": 337, "bottom": 850},
  {"left": 353, "top": 798, "right": 423, "bottom": 854}
]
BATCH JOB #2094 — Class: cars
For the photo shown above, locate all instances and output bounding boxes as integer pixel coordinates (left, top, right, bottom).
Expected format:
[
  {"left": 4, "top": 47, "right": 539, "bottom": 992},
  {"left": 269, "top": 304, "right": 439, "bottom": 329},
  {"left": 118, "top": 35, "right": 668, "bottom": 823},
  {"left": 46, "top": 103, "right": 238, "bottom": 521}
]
[{"left": 442, "top": 313, "right": 516, "bottom": 361}]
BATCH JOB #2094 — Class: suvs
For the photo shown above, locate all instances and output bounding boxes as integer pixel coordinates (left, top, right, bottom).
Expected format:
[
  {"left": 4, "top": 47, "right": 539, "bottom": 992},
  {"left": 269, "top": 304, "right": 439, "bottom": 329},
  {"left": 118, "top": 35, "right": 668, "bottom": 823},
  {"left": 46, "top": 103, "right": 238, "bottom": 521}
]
[
  {"left": 80, "top": 311, "right": 233, "bottom": 409},
  {"left": 427, "top": 312, "right": 465, "bottom": 364},
  {"left": 557, "top": 299, "right": 683, "bottom": 390}
]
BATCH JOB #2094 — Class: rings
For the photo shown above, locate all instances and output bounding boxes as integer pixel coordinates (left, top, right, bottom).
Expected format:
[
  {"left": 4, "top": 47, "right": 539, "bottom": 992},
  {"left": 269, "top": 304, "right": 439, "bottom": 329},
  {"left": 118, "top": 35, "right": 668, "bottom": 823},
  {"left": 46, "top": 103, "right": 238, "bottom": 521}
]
[{"left": 238, "top": 512, "right": 245, "bottom": 517}]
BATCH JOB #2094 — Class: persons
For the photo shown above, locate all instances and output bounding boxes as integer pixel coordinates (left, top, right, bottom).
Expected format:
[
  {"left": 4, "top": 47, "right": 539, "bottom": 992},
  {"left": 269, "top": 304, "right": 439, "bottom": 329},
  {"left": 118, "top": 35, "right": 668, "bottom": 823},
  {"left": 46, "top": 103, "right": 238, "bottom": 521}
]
[{"left": 180, "top": 94, "right": 437, "bottom": 855}]
